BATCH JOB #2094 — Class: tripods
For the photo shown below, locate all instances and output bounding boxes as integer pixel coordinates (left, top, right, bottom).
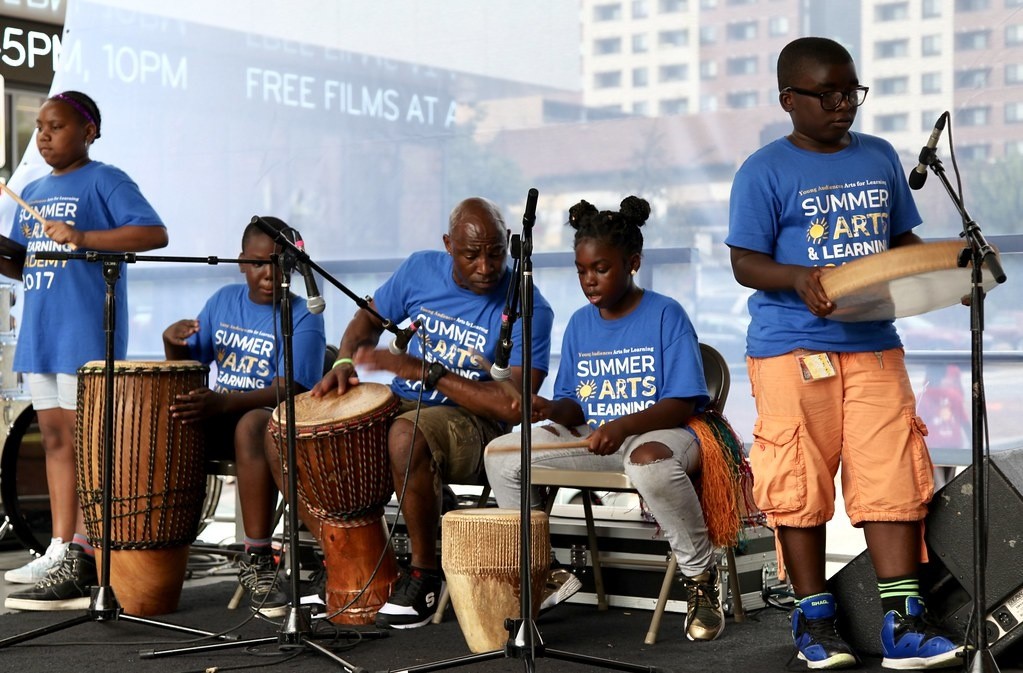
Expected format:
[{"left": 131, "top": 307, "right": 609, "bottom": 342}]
[{"left": 0, "top": 189, "right": 672, "bottom": 673}]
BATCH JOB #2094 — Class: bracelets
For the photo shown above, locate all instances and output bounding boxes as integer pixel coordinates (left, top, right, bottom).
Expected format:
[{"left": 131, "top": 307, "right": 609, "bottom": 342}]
[{"left": 331, "top": 357, "right": 356, "bottom": 368}]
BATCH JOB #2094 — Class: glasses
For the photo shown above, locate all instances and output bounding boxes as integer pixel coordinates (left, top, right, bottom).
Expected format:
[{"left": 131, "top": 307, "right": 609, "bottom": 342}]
[{"left": 783, "top": 80, "right": 869, "bottom": 111}]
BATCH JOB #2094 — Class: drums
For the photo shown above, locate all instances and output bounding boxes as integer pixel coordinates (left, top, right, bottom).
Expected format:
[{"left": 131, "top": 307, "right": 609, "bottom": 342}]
[
  {"left": 268, "top": 384, "right": 405, "bottom": 627},
  {"left": 1, "top": 396, "right": 32, "bottom": 449},
  {"left": 818, "top": 241, "right": 999, "bottom": 323},
  {"left": 73, "top": 359, "right": 210, "bottom": 619},
  {"left": 440, "top": 510, "right": 553, "bottom": 655},
  {"left": 0, "top": 402, "right": 225, "bottom": 561}
]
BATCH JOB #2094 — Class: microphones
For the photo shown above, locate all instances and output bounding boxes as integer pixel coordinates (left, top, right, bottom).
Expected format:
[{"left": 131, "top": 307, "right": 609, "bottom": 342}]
[
  {"left": 490, "top": 339, "right": 513, "bottom": 382},
  {"left": 910, "top": 114, "right": 947, "bottom": 189},
  {"left": 293, "top": 230, "right": 325, "bottom": 314}
]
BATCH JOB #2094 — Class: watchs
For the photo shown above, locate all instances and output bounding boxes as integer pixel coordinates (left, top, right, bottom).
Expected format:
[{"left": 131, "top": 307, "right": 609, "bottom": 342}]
[{"left": 425, "top": 362, "right": 447, "bottom": 392}]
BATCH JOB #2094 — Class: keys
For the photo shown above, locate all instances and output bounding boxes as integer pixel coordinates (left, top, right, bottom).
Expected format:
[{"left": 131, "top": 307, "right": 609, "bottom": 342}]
[{"left": 874, "top": 351, "right": 883, "bottom": 369}]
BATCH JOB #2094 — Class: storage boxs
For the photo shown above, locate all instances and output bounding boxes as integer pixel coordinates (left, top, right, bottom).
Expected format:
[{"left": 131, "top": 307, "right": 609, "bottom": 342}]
[{"left": 285, "top": 507, "right": 794, "bottom": 616}]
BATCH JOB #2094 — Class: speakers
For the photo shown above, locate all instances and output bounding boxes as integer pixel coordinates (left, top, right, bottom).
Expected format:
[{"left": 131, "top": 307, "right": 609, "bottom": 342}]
[{"left": 821, "top": 446, "right": 1023, "bottom": 668}]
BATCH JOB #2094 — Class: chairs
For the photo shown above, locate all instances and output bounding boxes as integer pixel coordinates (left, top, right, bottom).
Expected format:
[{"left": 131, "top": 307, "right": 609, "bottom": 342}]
[{"left": 201, "top": 342, "right": 745, "bottom": 644}]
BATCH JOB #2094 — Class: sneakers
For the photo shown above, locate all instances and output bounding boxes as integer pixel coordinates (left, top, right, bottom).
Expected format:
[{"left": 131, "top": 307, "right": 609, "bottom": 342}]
[
  {"left": 535, "top": 567, "right": 582, "bottom": 606},
  {"left": 679, "top": 562, "right": 726, "bottom": 642},
  {"left": 3, "top": 549, "right": 100, "bottom": 611},
  {"left": 878, "top": 596, "right": 974, "bottom": 669},
  {"left": 384, "top": 562, "right": 448, "bottom": 629},
  {"left": 236, "top": 545, "right": 290, "bottom": 618},
  {"left": 4, "top": 536, "right": 72, "bottom": 584},
  {"left": 783, "top": 592, "right": 855, "bottom": 670}
]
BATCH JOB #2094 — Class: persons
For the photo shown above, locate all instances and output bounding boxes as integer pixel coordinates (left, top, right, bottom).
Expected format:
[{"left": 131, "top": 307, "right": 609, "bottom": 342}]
[
  {"left": 299, "top": 195, "right": 555, "bottom": 623},
  {"left": 482, "top": 196, "right": 725, "bottom": 643},
  {"left": 0, "top": 91, "right": 169, "bottom": 586},
  {"left": 724, "top": 35, "right": 989, "bottom": 671},
  {"left": 4, "top": 215, "right": 327, "bottom": 617}
]
